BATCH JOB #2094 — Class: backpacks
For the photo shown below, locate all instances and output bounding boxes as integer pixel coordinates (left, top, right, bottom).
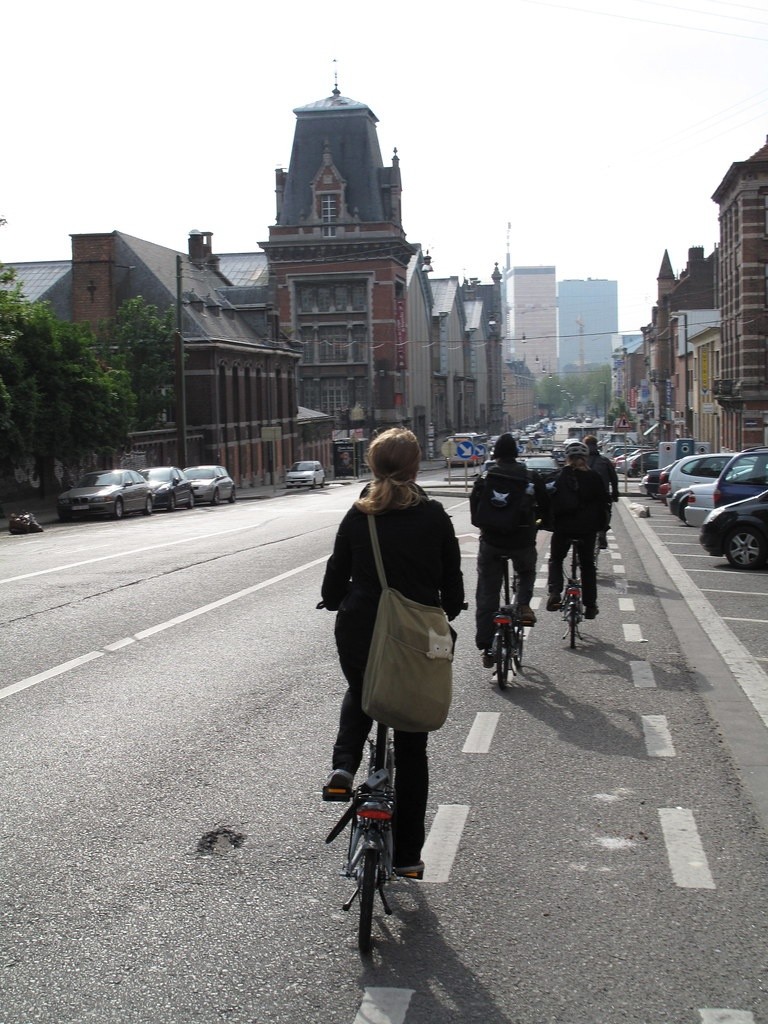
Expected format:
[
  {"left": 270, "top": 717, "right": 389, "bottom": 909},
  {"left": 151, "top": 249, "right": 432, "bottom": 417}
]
[{"left": 471, "top": 466, "right": 535, "bottom": 536}]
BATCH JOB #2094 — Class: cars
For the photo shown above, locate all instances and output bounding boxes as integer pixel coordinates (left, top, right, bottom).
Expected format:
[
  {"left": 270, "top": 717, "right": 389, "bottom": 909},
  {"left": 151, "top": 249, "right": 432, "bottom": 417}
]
[
  {"left": 137, "top": 467, "right": 196, "bottom": 514},
  {"left": 183, "top": 464, "right": 235, "bottom": 506},
  {"left": 713, "top": 447, "right": 768, "bottom": 507},
  {"left": 698, "top": 488, "right": 768, "bottom": 570},
  {"left": 489, "top": 416, "right": 555, "bottom": 455},
  {"left": 576, "top": 416, "right": 593, "bottom": 423},
  {"left": 554, "top": 431, "right": 658, "bottom": 477},
  {"left": 58, "top": 469, "right": 156, "bottom": 520},
  {"left": 284, "top": 460, "right": 324, "bottom": 490},
  {"left": 639, "top": 453, "right": 756, "bottom": 527}
]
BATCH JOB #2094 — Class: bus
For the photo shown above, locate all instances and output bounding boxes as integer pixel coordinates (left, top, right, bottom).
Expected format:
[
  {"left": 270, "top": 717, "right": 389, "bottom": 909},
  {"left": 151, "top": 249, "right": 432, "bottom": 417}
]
[
  {"left": 445, "top": 433, "right": 487, "bottom": 466},
  {"left": 567, "top": 426, "right": 613, "bottom": 442}
]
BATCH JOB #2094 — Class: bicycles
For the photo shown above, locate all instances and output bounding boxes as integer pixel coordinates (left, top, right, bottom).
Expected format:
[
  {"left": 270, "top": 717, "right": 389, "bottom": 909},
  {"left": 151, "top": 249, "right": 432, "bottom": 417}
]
[
  {"left": 555, "top": 539, "right": 586, "bottom": 644},
  {"left": 325, "top": 716, "right": 394, "bottom": 958},
  {"left": 487, "top": 551, "right": 524, "bottom": 682}
]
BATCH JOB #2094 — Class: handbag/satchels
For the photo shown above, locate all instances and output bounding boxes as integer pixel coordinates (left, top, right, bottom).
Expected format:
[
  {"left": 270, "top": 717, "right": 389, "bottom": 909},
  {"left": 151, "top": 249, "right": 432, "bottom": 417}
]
[{"left": 361, "top": 588, "right": 454, "bottom": 733}]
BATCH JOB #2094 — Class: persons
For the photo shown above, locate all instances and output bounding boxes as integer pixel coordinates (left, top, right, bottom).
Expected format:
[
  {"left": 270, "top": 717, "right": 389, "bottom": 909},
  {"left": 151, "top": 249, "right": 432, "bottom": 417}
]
[
  {"left": 470, "top": 432, "right": 553, "bottom": 650},
  {"left": 321, "top": 426, "right": 464, "bottom": 874},
  {"left": 538, "top": 435, "right": 618, "bottom": 618}
]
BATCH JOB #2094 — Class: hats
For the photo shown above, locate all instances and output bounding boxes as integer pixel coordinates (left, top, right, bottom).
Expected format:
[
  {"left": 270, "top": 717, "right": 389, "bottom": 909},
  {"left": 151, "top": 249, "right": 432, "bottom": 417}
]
[
  {"left": 494, "top": 434, "right": 519, "bottom": 459},
  {"left": 583, "top": 435, "right": 597, "bottom": 451}
]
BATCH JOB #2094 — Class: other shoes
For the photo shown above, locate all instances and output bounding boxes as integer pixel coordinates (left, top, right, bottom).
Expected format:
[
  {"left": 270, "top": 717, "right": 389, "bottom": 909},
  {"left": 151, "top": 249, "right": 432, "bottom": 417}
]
[
  {"left": 597, "top": 535, "right": 608, "bottom": 547},
  {"left": 546, "top": 590, "right": 561, "bottom": 611},
  {"left": 483, "top": 650, "right": 494, "bottom": 668},
  {"left": 324, "top": 768, "right": 353, "bottom": 791},
  {"left": 585, "top": 603, "right": 599, "bottom": 619},
  {"left": 395, "top": 861, "right": 424, "bottom": 875},
  {"left": 513, "top": 604, "right": 538, "bottom": 623}
]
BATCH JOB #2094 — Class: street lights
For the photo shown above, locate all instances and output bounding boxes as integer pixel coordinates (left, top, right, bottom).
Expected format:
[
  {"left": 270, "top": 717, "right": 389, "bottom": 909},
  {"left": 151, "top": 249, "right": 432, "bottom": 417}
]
[
  {"left": 599, "top": 382, "right": 607, "bottom": 427},
  {"left": 671, "top": 313, "right": 689, "bottom": 436}
]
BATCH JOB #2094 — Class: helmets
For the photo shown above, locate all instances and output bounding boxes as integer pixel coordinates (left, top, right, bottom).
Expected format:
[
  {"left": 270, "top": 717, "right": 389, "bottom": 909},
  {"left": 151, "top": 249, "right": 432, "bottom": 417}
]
[{"left": 565, "top": 441, "right": 590, "bottom": 457}]
